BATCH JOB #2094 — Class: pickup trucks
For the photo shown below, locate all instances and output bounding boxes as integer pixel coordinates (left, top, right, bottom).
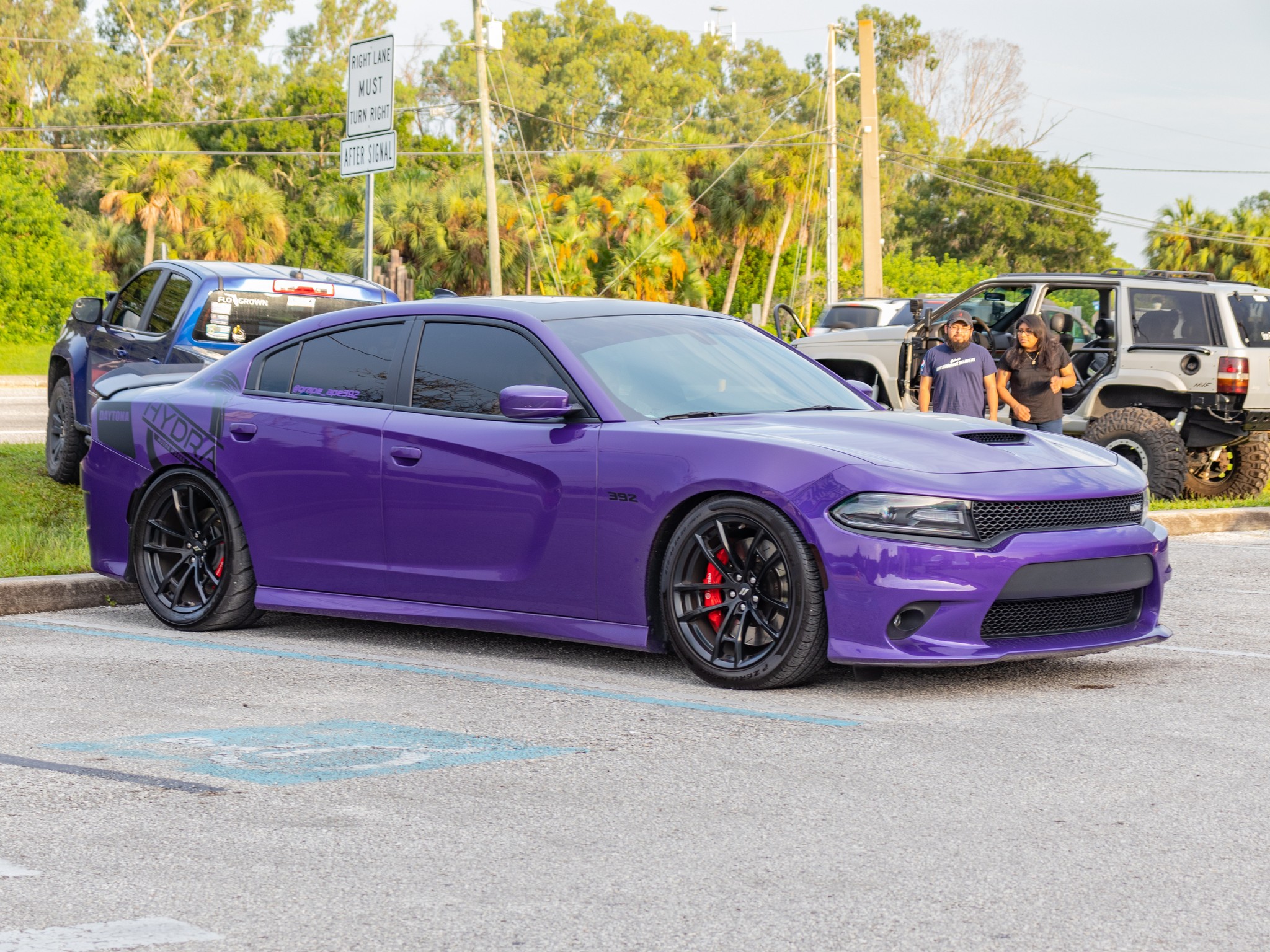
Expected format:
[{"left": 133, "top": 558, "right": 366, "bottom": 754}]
[{"left": 45, "top": 247, "right": 461, "bottom": 487}]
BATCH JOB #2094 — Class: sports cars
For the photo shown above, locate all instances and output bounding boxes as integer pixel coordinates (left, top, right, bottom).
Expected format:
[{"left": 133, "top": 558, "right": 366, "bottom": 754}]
[{"left": 79, "top": 294, "right": 1174, "bottom": 692}]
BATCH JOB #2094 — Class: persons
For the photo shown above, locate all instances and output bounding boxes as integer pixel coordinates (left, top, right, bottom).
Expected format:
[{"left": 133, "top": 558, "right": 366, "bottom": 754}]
[
  {"left": 997, "top": 314, "right": 1077, "bottom": 435},
  {"left": 918, "top": 309, "right": 998, "bottom": 421}
]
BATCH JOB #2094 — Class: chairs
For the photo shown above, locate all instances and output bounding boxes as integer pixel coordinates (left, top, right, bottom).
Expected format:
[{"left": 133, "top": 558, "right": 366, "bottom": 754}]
[
  {"left": 1176, "top": 319, "right": 1200, "bottom": 344},
  {"left": 1062, "top": 308, "right": 1179, "bottom": 408},
  {"left": 1070, "top": 317, "right": 1113, "bottom": 375},
  {"left": 1050, "top": 313, "right": 1074, "bottom": 355}
]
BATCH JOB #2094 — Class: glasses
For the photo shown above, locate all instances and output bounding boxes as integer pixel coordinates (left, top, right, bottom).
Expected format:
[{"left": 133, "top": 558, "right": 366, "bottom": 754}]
[
  {"left": 1016, "top": 329, "right": 1033, "bottom": 336},
  {"left": 948, "top": 324, "right": 972, "bottom": 333}
]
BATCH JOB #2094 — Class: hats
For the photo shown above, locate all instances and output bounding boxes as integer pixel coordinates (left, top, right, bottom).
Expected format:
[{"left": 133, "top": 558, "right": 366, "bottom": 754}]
[{"left": 947, "top": 309, "right": 973, "bottom": 326}]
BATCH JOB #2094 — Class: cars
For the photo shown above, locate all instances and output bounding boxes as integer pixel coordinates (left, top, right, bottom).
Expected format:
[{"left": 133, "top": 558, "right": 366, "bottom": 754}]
[
  {"left": 887, "top": 299, "right": 1014, "bottom": 353},
  {"left": 1008, "top": 302, "right": 1101, "bottom": 353}
]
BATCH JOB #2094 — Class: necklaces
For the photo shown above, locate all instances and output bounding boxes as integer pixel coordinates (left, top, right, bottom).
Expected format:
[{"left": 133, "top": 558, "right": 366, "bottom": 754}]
[{"left": 1024, "top": 351, "right": 1040, "bottom": 366}]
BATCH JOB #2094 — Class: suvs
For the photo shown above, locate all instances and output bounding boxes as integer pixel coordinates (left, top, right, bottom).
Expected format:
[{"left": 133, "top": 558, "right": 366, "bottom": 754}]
[
  {"left": 773, "top": 263, "right": 1269, "bottom": 503},
  {"left": 807, "top": 296, "right": 910, "bottom": 337}
]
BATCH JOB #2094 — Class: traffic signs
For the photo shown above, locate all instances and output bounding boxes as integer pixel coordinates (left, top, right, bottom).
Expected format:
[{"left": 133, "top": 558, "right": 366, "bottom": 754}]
[{"left": 339, "top": 34, "right": 396, "bottom": 178}]
